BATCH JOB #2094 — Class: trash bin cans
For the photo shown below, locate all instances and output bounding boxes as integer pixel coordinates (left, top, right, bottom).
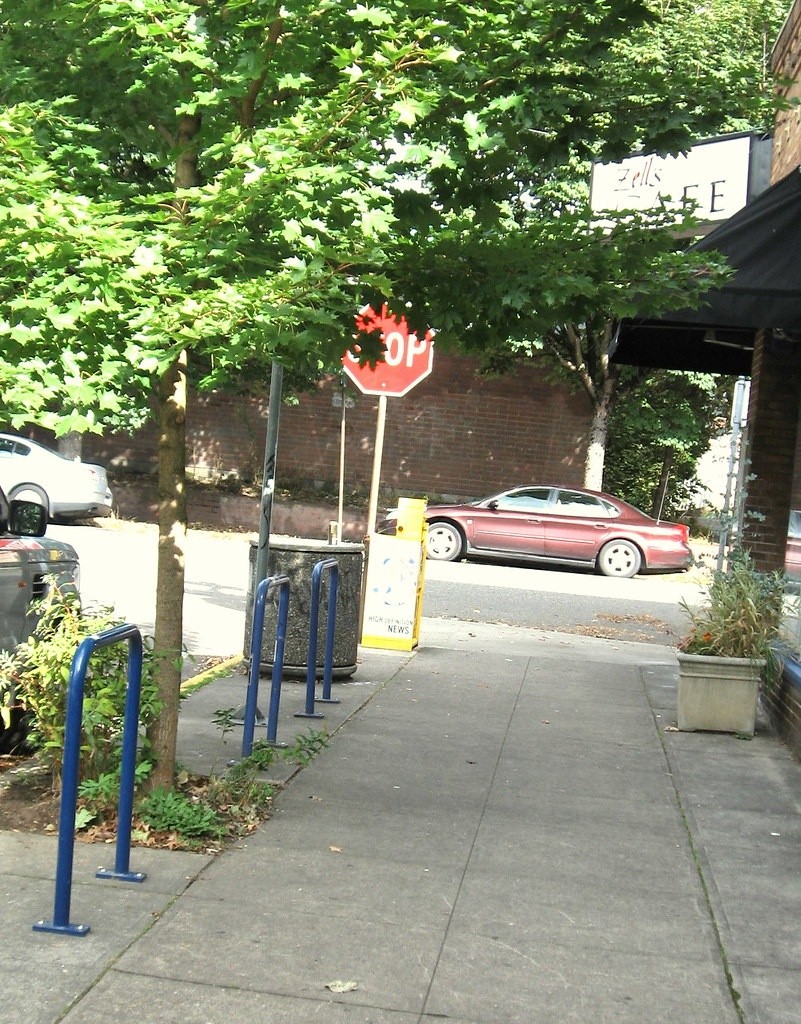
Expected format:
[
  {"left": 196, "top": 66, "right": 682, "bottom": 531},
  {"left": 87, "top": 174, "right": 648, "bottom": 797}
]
[{"left": 243, "top": 539, "right": 366, "bottom": 677}]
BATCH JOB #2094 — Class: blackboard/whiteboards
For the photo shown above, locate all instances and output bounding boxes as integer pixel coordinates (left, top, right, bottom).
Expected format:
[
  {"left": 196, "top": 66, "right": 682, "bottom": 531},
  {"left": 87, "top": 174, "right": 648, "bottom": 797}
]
[{"left": 362, "top": 535, "right": 422, "bottom": 640}]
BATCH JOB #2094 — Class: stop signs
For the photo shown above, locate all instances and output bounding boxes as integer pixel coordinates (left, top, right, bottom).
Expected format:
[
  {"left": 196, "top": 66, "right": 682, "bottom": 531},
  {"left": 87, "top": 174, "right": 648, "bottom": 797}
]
[{"left": 340, "top": 300, "right": 436, "bottom": 398}]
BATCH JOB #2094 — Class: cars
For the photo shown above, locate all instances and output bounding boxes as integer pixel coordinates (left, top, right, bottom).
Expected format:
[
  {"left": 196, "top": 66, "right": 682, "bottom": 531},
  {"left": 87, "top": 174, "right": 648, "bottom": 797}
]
[
  {"left": 385, "top": 484, "right": 694, "bottom": 578},
  {"left": 726, "top": 509, "right": 801, "bottom": 592},
  {"left": 0, "top": 433, "right": 113, "bottom": 757}
]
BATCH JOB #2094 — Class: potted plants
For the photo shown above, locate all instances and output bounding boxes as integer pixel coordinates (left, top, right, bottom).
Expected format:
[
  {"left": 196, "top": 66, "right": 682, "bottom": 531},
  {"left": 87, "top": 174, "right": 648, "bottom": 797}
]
[{"left": 666, "top": 546, "right": 801, "bottom": 739}]
